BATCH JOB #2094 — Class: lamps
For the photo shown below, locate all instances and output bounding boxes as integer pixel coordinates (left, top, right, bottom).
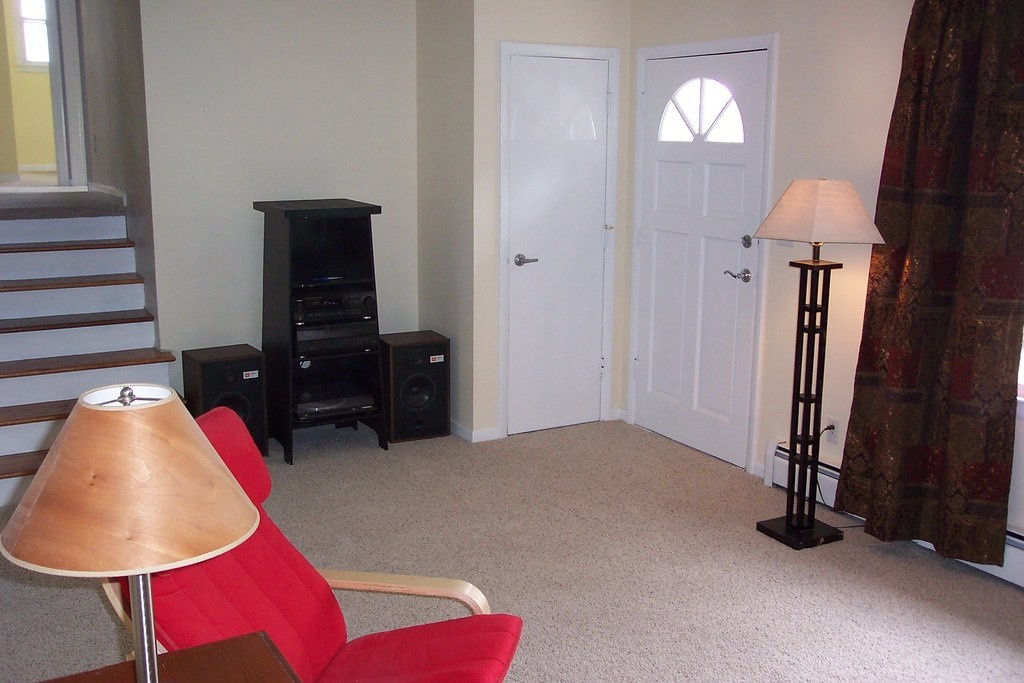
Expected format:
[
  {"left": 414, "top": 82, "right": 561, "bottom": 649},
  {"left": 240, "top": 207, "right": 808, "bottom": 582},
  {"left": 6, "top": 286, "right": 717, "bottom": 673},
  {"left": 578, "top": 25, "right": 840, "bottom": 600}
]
[
  {"left": 753, "top": 177, "right": 886, "bottom": 550},
  {"left": 0, "top": 382, "right": 261, "bottom": 683}
]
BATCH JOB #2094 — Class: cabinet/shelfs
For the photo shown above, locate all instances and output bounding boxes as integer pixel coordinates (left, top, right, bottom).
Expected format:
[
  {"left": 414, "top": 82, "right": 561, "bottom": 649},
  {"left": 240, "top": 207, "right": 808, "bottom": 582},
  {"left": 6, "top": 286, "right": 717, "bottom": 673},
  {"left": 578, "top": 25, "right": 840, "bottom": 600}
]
[{"left": 253, "top": 198, "right": 389, "bottom": 465}]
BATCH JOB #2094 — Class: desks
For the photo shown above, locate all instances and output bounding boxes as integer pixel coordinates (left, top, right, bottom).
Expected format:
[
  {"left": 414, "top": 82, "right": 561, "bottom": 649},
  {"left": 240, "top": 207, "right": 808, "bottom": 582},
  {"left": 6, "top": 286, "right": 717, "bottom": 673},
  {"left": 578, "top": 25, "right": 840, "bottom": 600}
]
[{"left": 39, "top": 631, "right": 305, "bottom": 683}]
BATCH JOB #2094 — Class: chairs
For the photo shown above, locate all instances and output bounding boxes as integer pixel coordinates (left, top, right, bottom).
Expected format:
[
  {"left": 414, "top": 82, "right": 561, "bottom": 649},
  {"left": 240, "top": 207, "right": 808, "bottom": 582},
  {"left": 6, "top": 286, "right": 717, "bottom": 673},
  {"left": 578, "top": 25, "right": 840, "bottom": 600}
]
[{"left": 98, "top": 407, "right": 522, "bottom": 683}]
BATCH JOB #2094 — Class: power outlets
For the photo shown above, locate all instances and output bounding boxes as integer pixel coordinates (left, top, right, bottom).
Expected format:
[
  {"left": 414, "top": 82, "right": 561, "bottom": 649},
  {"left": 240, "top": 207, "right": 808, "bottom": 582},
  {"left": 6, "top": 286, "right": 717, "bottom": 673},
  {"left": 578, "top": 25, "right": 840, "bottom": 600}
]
[{"left": 826, "top": 417, "right": 840, "bottom": 445}]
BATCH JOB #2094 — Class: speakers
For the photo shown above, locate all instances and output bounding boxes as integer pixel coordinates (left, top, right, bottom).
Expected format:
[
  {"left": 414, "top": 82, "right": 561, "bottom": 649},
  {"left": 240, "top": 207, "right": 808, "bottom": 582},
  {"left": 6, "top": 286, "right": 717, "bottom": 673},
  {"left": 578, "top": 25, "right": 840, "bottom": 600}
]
[
  {"left": 183, "top": 344, "right": 268, "bottom": 458},
  {"left": 379, "top": 329, "right": 452, "bottom": 444}
]
